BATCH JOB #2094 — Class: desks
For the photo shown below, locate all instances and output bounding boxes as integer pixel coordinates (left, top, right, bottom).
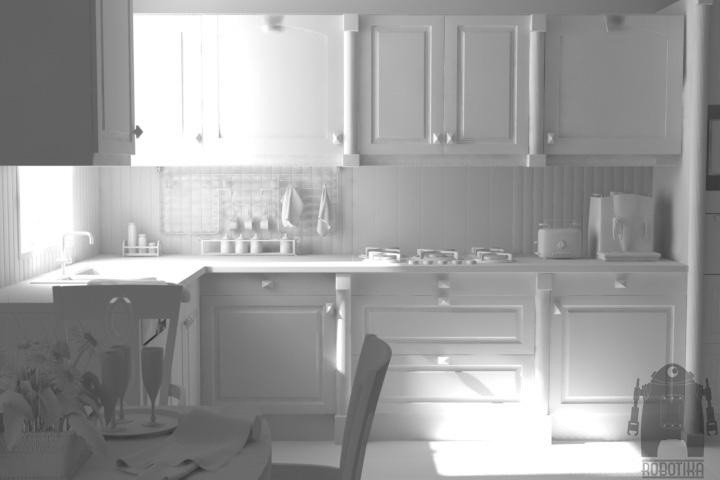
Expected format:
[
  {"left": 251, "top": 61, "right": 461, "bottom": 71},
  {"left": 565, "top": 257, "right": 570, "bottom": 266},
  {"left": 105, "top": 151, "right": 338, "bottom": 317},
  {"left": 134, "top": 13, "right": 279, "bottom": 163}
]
[{"left": 34, "top": 405, "right": 272, "bottom": 480}]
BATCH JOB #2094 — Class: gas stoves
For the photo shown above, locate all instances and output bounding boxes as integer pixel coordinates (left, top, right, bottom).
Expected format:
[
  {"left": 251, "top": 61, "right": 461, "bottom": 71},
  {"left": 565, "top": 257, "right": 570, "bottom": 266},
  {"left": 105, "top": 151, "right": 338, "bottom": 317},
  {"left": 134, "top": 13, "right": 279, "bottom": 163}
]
[{"left": 357, "top": 243, "right": 514, "bottom": 269}]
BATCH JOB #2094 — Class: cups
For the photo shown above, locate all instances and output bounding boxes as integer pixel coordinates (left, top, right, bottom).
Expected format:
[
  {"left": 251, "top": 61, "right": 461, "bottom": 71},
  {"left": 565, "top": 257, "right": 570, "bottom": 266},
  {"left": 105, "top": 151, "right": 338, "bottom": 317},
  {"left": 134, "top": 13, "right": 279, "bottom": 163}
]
[{"left": 126, "top": 221, "right": 156, "bottom": 254}]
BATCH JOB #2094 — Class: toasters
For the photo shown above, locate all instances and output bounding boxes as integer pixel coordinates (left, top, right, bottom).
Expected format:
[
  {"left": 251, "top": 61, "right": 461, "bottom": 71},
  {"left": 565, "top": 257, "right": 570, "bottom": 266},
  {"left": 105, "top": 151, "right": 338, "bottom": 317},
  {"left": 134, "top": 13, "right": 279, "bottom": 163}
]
[{"left": 534, "top": 223, "right": 583, "bottom": 260}]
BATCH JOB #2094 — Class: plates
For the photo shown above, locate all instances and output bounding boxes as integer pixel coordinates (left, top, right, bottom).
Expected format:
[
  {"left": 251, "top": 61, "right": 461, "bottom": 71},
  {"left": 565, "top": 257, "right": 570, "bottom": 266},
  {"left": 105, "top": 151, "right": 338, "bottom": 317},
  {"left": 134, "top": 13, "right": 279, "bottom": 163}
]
[{"left": 94, "top": 412, "right": 180, "bottom": 440}]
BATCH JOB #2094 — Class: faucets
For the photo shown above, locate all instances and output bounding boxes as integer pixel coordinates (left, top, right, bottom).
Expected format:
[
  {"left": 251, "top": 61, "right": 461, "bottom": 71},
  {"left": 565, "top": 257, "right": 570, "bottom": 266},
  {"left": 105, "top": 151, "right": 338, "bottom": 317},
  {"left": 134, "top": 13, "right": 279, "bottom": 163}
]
[{"left": 60, "top": 230, "right": 95, "bottom": 280}]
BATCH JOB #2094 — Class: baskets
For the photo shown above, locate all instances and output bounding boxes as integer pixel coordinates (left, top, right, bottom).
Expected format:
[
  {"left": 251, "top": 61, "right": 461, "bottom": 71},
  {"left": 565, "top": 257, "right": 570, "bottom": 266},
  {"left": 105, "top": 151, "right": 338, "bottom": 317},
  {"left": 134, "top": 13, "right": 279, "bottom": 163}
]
[{"left": 0, "top": 431, "right": 91, "bottom": 480}]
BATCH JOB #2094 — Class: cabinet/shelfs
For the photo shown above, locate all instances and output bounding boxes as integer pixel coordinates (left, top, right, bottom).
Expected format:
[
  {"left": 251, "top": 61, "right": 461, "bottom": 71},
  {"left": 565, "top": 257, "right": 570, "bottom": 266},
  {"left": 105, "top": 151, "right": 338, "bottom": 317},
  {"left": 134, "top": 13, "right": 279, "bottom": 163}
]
[
  {"left": 344, "top": 10, "right": 546, "bottom": 165},
  {"left": 0, "top": 1, "right": 135, "bottom": 167},
  {"left": 137, "top": 13, "right": 348, "bottom": 166},
  {"left": 541, "top": 9, "right": 680, "bottom": 157},
  {"left": 0, "top": 269, "right": 687, "bottom": 443}
]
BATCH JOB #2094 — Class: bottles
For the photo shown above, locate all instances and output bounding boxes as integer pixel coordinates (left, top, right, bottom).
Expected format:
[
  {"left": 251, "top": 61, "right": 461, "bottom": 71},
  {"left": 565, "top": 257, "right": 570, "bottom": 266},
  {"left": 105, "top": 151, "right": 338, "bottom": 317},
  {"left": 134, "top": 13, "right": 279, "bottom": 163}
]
[{"left": 220, "top": 233, "right": 293, "bottom": 255}]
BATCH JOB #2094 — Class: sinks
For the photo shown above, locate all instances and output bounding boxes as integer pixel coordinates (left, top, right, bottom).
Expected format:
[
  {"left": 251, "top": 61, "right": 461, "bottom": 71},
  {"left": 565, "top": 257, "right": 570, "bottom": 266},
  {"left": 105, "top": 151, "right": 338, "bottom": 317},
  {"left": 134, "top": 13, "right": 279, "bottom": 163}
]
[{"left": 76, "top": 263, "right": 189, "bottom": 277}]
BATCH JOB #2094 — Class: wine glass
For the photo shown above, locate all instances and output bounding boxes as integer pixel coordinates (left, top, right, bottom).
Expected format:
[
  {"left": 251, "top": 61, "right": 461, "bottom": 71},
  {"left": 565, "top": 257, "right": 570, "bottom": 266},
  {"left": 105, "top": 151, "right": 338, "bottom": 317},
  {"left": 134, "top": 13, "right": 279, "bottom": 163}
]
[
  {"left": 101, "top": 343, "right": 133, "bottom": 430},
  {"left": 141, "top": 345, "right": 164, "bottom": 428}
]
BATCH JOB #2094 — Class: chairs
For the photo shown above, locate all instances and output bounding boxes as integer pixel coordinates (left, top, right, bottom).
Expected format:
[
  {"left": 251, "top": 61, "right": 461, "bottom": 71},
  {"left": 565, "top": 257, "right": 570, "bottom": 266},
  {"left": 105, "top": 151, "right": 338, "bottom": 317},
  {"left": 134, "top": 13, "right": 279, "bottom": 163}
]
[{"left": 273, "top": 335, "right": 392, "bottom": 480}]
